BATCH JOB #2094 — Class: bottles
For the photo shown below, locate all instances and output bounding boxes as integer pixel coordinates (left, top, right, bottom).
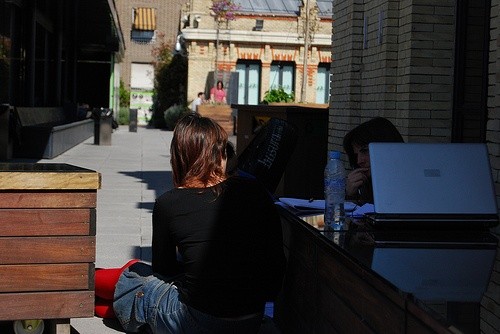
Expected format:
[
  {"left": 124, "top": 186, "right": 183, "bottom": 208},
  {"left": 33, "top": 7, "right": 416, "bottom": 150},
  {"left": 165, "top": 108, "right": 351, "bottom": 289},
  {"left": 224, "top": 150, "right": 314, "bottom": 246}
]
[{"left": 323, "top": 151, "right": 346, "bottom": 232}]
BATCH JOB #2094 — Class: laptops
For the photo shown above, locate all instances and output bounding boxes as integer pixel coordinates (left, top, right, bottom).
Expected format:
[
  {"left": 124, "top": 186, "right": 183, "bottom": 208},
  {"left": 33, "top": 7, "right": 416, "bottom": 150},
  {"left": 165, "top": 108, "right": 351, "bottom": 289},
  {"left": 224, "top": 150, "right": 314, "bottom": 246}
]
[{"left": 359, "top": 139, "right": 500, "bottom": 226}]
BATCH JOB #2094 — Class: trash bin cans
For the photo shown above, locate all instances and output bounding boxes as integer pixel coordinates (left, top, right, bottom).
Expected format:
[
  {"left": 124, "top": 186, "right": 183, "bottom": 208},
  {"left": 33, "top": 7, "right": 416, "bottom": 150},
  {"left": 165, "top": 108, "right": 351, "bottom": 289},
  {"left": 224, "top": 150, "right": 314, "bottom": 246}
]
[
  {"left": 94, "top": 107, "right": 112, "bottom": 146},
  {"left": 129, "top": 108, "right": 138, "bottom": 132}
]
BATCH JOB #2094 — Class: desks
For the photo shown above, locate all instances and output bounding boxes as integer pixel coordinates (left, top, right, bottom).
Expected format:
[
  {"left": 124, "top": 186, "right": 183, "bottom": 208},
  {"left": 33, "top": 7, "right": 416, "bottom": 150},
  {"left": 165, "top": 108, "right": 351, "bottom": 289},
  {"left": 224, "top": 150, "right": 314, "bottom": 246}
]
[{"left": 272, "top": 201, "right": 479, "bottom": 334}]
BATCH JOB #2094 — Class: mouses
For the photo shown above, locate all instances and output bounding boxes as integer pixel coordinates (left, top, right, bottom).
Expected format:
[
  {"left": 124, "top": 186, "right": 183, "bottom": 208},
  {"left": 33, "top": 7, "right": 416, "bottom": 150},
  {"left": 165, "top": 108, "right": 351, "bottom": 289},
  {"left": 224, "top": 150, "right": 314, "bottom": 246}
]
[{"left": 350, "top": 219, "right": 371, "bottom": 231}]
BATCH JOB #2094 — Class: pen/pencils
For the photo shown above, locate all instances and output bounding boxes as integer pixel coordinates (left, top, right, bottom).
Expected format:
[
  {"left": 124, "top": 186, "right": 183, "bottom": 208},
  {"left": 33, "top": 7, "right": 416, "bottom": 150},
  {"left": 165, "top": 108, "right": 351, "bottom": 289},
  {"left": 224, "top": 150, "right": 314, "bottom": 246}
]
[{"left": 308, "top": 198, "right": 314, "bottom": 204}]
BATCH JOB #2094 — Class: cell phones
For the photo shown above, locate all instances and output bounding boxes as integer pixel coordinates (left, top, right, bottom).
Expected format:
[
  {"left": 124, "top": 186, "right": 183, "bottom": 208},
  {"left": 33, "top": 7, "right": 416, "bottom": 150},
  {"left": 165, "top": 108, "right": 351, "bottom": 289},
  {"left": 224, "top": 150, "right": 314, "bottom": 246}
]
[{"left": 263, "top": 301, "right": 275, "bottom": 321}]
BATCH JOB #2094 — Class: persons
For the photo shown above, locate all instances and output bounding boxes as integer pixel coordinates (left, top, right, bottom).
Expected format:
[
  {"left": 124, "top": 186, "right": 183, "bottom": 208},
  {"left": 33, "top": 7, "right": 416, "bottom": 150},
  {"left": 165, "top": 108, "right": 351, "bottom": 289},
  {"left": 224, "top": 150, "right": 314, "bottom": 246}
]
[
  {"left": 343, "top": 117, "right": 405, "bottom": 203},
  {"left": 192, "top": 92, "right": 208, "bottom": 112},
  {"left": 94, "top": 113, "right": 284, "bottom": 334},
  {"left": 210, "top": 81, "right": 226, "bottom": 104}
]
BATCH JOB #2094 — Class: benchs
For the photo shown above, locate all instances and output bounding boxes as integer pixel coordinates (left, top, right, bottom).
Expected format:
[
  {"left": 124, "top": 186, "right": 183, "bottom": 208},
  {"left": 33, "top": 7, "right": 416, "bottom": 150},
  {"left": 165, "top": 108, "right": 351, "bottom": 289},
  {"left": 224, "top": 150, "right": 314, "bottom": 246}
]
[{"left": 12, "top": 107, "right": 95, "bottom": 159}]
